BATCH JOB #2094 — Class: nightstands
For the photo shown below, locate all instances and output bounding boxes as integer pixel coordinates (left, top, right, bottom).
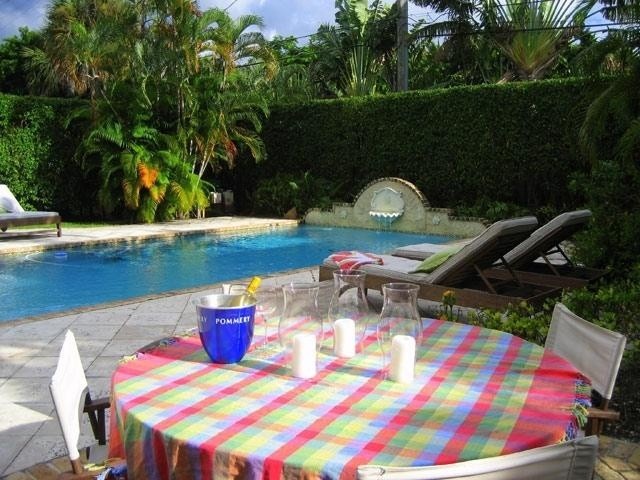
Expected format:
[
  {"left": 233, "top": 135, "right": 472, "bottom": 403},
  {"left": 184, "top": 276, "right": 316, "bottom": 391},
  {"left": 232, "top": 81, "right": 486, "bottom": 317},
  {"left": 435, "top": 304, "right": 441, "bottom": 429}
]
[{"left": 542, "top": 303, "right": 629, "bottom": 468}]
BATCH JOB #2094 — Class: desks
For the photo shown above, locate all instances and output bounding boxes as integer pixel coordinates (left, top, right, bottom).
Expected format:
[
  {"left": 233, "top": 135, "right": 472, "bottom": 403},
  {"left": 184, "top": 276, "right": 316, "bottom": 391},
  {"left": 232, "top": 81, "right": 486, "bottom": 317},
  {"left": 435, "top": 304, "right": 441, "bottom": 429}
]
[{"left": 112, "top": 311, "right": 591, "bottom": 480}]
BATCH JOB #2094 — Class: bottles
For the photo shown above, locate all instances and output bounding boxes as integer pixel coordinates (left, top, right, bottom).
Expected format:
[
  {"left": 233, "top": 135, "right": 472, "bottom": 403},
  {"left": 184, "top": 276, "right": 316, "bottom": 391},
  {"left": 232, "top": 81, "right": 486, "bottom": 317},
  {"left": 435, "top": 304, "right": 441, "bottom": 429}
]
[
  {"left": 225, "top": 275, "right": 261, "bottom": 310},
  {"left": 279, "top": 267, "right": 423, "bottom": 384}
]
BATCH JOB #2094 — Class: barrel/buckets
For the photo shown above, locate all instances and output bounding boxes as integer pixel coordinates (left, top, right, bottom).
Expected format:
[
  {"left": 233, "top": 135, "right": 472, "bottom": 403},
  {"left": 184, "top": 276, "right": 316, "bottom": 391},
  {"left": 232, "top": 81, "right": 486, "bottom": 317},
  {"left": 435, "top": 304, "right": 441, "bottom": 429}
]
[{"left": 190, "top": 291, "right": 259, "bottom": 365}]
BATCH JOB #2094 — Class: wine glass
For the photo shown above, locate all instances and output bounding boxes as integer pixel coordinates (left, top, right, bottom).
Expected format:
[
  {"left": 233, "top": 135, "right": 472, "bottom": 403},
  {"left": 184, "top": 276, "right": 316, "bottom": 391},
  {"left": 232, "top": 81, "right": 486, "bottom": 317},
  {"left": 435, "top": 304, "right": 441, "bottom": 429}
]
[{"left": 255, "top": 286, "right": 280, "bottom": 351}]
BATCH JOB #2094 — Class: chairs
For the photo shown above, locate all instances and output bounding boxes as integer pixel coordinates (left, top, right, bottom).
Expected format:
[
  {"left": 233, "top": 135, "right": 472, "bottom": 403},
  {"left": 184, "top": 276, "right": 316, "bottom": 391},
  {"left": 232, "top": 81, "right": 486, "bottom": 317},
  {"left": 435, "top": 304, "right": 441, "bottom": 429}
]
[
  {"left": 318, "top": 208, "right": 610, "bottom": 320},
  {"left": 355, "top": 435, "right": 600, "bottom": 479},
  {"left": 0, "top": 183, "right": 62, "bottom": 239},
  {"left": 47, "top": 330, "right": 129, "bottom": 480}
]
